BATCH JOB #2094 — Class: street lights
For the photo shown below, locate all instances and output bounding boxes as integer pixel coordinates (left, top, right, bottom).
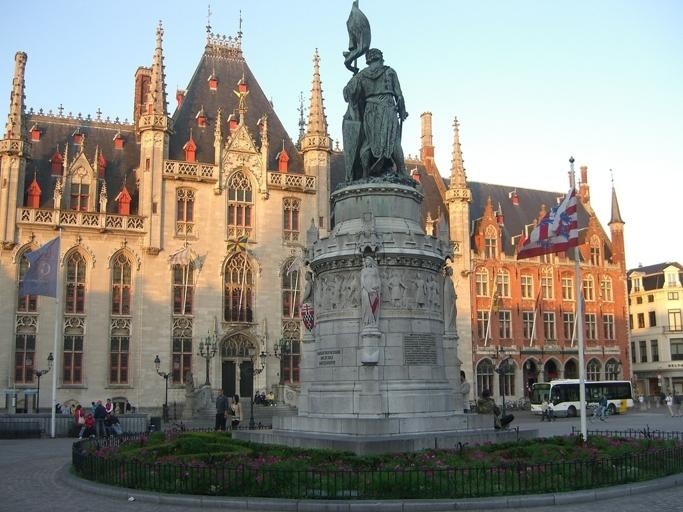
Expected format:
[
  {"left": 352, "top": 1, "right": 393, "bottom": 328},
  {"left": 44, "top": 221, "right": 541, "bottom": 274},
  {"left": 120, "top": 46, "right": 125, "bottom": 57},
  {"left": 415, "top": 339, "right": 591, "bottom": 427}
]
[
  {"left": 491, "top": 347, "right": 513, "bottom": 416},
  {"left": 26, "top": 353, "right": 54, "bottom": 412},
  {"left": 273, "top": 335, "right": 290, "bottom": 385},
  {"left": 154, "top": 355, "right": 180, "bottom": 423},
  {"left": 237, "top": 344, "right": 266, "bottom": 430},
  {"left": 199, "top": 334, "right": 219, "bottom": 385}
]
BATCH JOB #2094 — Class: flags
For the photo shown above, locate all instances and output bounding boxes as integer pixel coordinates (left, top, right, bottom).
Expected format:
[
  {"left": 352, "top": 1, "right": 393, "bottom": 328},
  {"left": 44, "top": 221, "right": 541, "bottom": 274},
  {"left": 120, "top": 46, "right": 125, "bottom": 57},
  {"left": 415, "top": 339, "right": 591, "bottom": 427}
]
[
  {"left": 16, "top": 236, "right": 59, "bottom": 301},
  {"left": 516, "top": 185, "right": 579, "bottom": 261}
]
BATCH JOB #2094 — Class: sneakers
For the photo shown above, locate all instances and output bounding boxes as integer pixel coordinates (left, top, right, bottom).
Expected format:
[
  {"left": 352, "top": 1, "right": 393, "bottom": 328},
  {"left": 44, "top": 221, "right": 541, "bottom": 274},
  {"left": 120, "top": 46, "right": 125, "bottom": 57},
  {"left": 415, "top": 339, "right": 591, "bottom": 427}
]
[
  {"left": 78, "top": 436, "right": 83, "bottom": 440},
  {"left": 89, "top": 434, "right": 95, "bottom": 438}
]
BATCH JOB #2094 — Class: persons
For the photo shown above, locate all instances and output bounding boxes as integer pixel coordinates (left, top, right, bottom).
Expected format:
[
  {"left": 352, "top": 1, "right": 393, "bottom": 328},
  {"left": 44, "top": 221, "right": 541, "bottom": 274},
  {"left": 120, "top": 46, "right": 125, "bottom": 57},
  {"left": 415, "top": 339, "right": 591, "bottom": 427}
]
[
  {"left": 460, "top": 370, "right": 471, "bottom": 414},
  {"left": 229, "top": 394, "right": 244, "bottom": 428},
  {"left": 253, "top": 391, "right": 277, "bottom": 406},
  {"left": 475, "top": 388, "right": 515, "bottom": 430},
  {"left": 215, "top": 388, "right": 228, "bottom": 433},
  {"left": 665, "top": 391, "right": 673, "bottom": 417},
  {"left": 638, "top": 393, "right": 645, "bottom": 414},
  {"left": 595, "top": 390, "right": 607, "bottom": 424},
  {"left": 59, "top": 394, "right": 130, "bottom": 442},
  {"left": 546, "top": 398, "right": 555, "bottom": 422},
  {"left": 343, "top": 47, "right": 411, "bottom": 186},
  {"left": 539, "top": 397, "right": 548, "bottom": 423}
]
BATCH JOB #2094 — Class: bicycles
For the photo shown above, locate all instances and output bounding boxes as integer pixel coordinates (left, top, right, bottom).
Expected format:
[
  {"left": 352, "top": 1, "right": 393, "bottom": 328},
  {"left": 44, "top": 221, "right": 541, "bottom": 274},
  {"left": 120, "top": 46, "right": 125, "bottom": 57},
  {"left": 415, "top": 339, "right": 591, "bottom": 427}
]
[{"left": 505, "top": 399, "right": 528, "bottom": 410}]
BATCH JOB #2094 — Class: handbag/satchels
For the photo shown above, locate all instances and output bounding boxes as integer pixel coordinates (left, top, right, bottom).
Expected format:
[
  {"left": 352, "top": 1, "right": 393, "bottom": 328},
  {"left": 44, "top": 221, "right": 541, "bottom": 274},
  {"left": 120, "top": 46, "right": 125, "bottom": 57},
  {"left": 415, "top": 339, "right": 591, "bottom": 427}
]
[
  {"left": 78, "top": 410, "right": 85, "bottom": 425},
  {"left": 227, "top": 406, "right": 235, "bottom": 417}
]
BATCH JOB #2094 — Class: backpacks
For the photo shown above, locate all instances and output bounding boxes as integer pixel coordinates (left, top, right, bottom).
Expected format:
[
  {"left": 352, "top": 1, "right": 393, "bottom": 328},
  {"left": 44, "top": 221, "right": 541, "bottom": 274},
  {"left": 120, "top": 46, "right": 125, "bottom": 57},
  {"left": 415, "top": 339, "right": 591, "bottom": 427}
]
[{"left": 105, "top": 415, "right": 122, "bottom": 434}]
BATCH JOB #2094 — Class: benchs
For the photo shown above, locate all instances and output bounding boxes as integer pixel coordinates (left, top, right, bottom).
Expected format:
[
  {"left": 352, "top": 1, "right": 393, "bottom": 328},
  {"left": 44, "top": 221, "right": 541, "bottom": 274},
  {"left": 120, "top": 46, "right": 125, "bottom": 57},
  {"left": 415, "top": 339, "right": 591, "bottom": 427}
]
[
  {"left": 0, "top": 422, "right": 43, "bottom": 439},
  {"left": 68, "top": 421, "right": 77, "bottom": 437}
]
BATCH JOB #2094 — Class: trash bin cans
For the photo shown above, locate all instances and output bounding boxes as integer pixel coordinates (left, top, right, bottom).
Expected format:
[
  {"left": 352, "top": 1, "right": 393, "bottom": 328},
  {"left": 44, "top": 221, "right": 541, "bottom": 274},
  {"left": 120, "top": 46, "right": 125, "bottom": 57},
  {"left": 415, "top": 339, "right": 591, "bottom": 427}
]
[
  {"left": 151, "top": 417, "right": 161, "bottom": 431},
  {"left": 6, "top": 390, "right": 16, "bottom": 414},
  {"left": 25, "top": 390, "right": 35, "bottom": 413}
]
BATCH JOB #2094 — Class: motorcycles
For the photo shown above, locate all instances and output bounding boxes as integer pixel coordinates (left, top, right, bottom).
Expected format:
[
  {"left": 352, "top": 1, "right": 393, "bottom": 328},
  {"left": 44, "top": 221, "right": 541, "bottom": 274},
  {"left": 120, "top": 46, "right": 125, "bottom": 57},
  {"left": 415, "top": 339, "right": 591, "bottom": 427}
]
[{"left": 89, "top": 410, "right": 122, "bottom": 438}]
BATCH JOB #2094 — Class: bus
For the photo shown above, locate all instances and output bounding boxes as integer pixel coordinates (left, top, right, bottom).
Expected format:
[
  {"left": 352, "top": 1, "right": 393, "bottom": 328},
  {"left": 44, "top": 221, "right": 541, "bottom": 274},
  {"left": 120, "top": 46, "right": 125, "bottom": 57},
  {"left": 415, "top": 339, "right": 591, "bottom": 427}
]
[{"left": 531, "top": 379, "right": 634, "bottom": 418}]
